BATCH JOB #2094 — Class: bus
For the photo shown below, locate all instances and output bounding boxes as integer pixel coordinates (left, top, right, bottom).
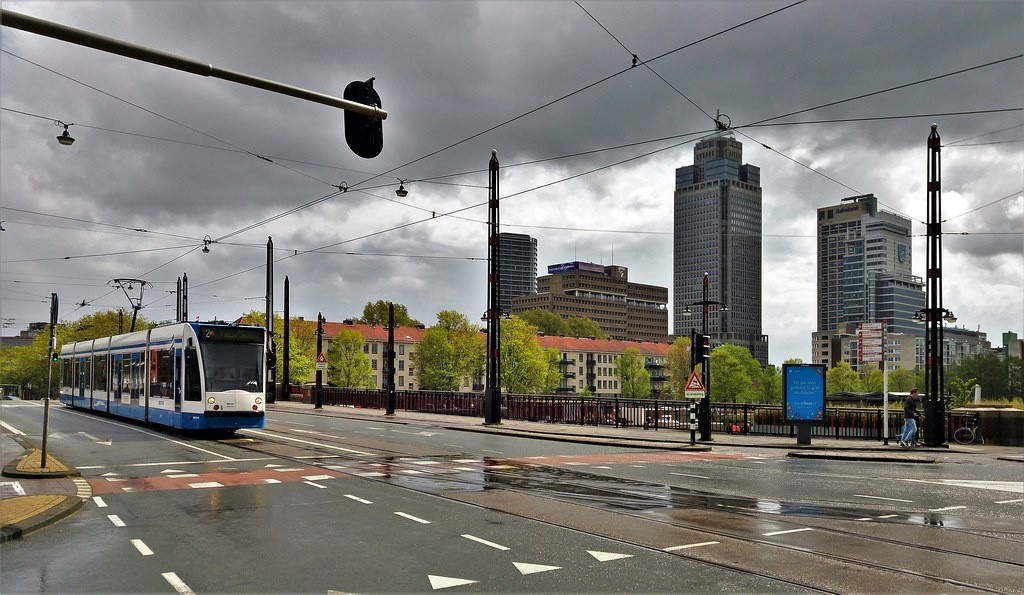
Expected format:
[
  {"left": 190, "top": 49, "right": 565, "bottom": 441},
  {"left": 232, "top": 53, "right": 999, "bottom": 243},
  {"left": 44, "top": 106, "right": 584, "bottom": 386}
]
[{"left": 59, "top": 278, "right": 277, "bottom": 436}]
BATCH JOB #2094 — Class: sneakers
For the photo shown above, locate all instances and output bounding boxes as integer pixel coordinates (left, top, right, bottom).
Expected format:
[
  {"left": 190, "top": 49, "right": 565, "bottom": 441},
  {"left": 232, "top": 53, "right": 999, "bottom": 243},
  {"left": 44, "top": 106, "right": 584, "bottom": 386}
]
[
  {"left": 900, "top": 443, "right": 905, "bottom": 447},
  {"left": 902, "top": 441, "right": 910, "bottom": 448}
]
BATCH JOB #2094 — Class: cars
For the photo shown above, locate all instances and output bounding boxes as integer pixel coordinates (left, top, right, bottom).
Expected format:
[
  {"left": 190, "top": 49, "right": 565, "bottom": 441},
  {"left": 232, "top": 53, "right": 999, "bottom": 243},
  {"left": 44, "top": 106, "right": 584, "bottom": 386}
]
[{"left": 3, "top": 395, "right": 21, "bottom": 400}]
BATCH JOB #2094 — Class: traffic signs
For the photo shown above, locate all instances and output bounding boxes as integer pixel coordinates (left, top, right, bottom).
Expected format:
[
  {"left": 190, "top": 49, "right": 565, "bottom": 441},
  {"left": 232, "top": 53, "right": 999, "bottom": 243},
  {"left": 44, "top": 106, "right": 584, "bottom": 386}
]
[
  {"left": 859, "top": 339, "right": 882, "bottom": 345},
  {"left": 859, "top": 354, "right": 883, "bottom": 362},
  {"left": 859, "top": 347, "right": 883, "bottom": 353},
  {"left": 858, "top": 331, "right": 882, "bottom": 339},
  {"left": 859, "top": 323, "right": 883, "bottom": 330}
]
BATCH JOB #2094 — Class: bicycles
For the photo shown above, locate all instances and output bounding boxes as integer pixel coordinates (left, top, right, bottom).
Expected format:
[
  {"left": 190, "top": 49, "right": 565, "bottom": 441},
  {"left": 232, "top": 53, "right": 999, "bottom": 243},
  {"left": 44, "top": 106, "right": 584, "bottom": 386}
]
[{"left": 954, "top": 418, "right": 985, "bottom": 445}]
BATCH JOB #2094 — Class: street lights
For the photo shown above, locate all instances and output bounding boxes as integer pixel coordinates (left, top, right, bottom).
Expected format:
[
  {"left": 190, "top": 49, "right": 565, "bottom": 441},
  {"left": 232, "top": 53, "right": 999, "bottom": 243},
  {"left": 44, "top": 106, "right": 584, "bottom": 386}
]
[
  {"left": 682, "top": 272, "right": 730, "bottom": 441},
  {"left": 313, "top": 312, "right": 327, "bottom": 408},
  {"left": 382, "top": 301, "right": 399, "bottom": 416}
]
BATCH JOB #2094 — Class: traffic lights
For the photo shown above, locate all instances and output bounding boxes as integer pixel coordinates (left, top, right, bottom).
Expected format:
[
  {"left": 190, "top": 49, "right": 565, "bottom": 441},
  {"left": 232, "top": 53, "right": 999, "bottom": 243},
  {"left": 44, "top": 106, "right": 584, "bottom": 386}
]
[
  {"left": 53, "top": 353, "right": 58, "bottom": 362},
  {"left": 693, "top": 333, "right": 711, "bottom": 365}
]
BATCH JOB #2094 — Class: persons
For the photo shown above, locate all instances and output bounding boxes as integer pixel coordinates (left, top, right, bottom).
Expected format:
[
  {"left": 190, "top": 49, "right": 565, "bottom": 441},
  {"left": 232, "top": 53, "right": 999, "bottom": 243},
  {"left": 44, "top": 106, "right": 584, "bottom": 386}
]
[
  {"left": 896, "top": 389, "right": 926, "bottom": 448},
  {"left": 211, "top": 366, "right": 232, "bottom": 380}
]
